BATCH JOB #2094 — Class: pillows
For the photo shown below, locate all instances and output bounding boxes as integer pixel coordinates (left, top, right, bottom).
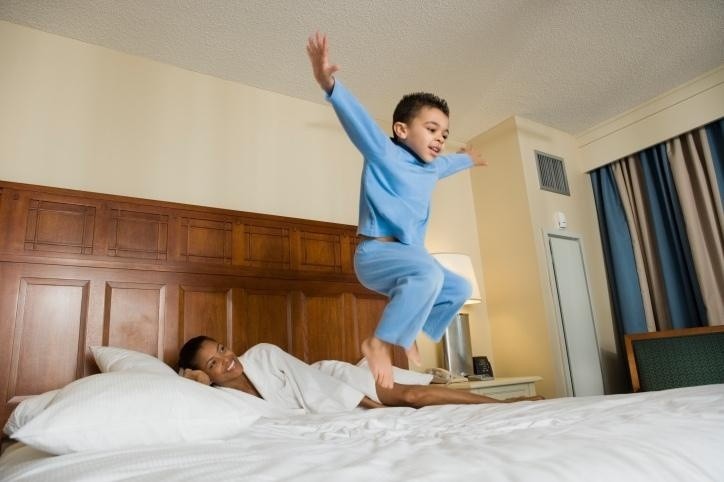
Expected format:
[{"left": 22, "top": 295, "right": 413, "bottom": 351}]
[{"left": 3, "top": 344, "right": 273, "bottom": 457}]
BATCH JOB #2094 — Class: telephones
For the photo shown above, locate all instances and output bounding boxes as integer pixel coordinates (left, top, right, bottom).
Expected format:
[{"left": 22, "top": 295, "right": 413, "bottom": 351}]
[{"left": 425, "top": 368, "right": 468, "bottom": 384}]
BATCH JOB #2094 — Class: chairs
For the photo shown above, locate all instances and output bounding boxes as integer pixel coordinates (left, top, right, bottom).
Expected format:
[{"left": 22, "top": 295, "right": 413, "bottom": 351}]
[{"left": 624, "top": 324, "right": 723, "bottom": 395}]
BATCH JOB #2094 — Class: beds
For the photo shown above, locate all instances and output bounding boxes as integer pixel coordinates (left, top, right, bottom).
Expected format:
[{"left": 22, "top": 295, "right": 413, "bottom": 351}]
[{"left": 1, "top": 381, "right": 724, "bottom": 482}]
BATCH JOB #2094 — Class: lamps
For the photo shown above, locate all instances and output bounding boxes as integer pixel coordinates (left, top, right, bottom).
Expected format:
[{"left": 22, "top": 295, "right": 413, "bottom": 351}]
[{"left": 428, "top": 252, "right": 484, "bottom": 380}]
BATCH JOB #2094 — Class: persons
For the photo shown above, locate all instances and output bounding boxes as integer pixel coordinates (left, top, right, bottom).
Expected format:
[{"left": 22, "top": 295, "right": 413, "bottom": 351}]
[
  {"left": 304, "top": 29, "right": 492, "bottom": 392},
  {"left": 175, "top": 334, "right": 546, "bottom": 411}
]
